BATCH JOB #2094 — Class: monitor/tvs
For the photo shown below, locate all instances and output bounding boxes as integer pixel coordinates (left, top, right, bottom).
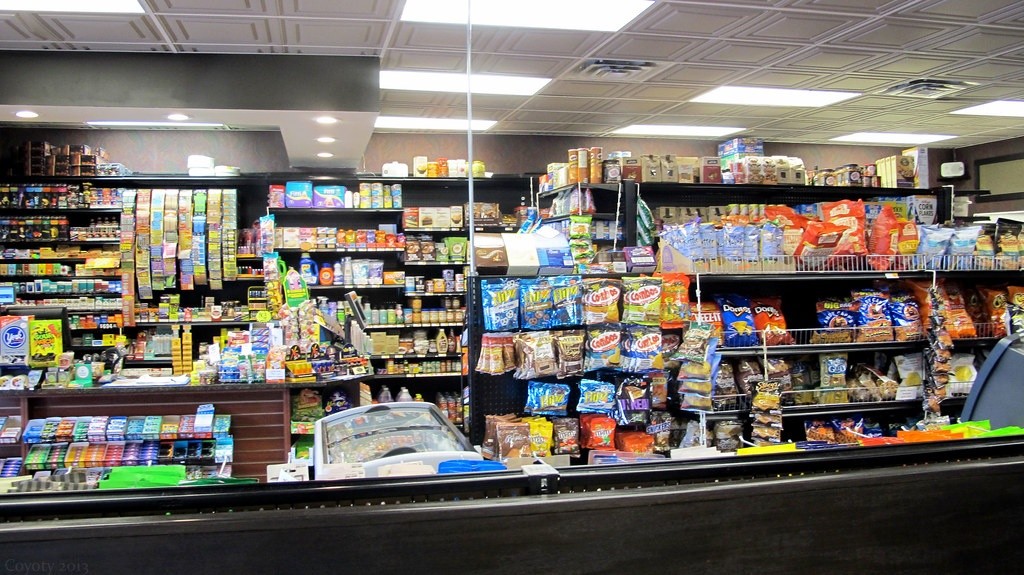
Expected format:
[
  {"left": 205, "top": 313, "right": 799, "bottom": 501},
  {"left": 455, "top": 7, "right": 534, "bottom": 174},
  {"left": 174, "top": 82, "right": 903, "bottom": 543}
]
[{"left": 960, "top": 332, "right": 1024, "bottom": 429}]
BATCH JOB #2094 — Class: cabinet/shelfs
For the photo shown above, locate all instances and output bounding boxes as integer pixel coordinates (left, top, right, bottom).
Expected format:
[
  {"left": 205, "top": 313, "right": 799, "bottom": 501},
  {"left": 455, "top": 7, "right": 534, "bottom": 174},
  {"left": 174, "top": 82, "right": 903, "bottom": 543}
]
[
  {"left": 261, "top": 172, "right": 546, "bottom": 480},
  {"left": 684, "top": 254, "right": 1024, "bottom": 446},
  {"left": 640, "top": 182, "right": 944, "bottom": 253},
  {"left": 940, "top": 185, "right": 991, "bottom": 225},
  {"left": 0, "top": 175, "right": 268, "bottom": 368},
  {"left": 538, "top": 179, "right": 638, "bottom": 246}
]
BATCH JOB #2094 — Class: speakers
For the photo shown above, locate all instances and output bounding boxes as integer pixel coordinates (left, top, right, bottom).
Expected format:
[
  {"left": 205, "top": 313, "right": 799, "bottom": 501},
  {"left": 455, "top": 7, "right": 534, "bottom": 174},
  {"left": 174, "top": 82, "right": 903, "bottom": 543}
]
[{"left": 941, "top": 161, "right": 965, "bottom": 178}]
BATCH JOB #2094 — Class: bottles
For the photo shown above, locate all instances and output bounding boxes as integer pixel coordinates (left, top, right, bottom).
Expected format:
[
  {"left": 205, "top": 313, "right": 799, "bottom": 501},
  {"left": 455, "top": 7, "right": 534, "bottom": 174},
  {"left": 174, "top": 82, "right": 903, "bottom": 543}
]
[{"left": 377, "top": 385, "right": 424, "bottom": 402}]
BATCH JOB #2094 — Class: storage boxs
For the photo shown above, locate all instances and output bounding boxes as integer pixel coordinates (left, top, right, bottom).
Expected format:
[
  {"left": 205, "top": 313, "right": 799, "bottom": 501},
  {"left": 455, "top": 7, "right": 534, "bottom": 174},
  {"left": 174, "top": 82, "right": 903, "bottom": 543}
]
[
  {"left": 721, "top": 153, "right": 763, "bottom": 171},
  {"left": 421, "top": 241, "right": 436, "bottom": 261},
  {"left": 533, "top": 233, "right": 575, "bottom": 276},
  {"left": 419, "top": 207, "right": 436, "bottom": 228},
  {"left": 501, "top": 233, "right": 540, "bottom": 276},
  {"left": 405, "top": 241, "right": 423, "bottom": 261},
  {"left": 0, "top": 364, "right": 370, "bottom": 481},
  {"left": 30, "top": 319, "right": 63, "bottom": 367},
  {"left": 717, "top": 137, "right": 764, "bottom": 158},
  {"left": 902, "top": 145, "right": 929, "bottom": 189},
  {"left": 905, "top": 194, "right": 938, "bottom": 225},
  {"left": 0, "top": 315, "right": 35, "bottom": 366},
  {"left": 9, "top": 141, "right": 133, "bottom": 176},
  {"left": 595, "top": 251, "right": 627, "bottom": 273},
  {"left": 413, "top": 156, "right": 428, "bottom": 177},
  {"left": 435, "top": 207, "right": 451, "bottom": 228},
  {"left": 384, "top": 271, "right": 405, "bottom": 285},
  {"left": 450, "top": 206, "right": 463, "bottom": 228},
  {"left": 463, "top": 232, "right": 509, "bottom": 276},
  {"left": 401, "top": 207, "right": 419, "bottom": 229},
  {"left": 622, "top": 246, "right": 658, "bottom": 273},
  {"left": 876, "top": 154, "right": 915, "bottom": 189},
  {"left": 698, "top": 157, "right": 722, "bottom": 184}
]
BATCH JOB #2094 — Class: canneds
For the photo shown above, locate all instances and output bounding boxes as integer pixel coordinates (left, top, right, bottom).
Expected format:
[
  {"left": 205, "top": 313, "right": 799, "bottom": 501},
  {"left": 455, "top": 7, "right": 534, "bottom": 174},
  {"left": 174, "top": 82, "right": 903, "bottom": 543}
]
[
  {"left": 816, "top": 162, "right": 882, "bottom": 186},
  {"left": 372, "top": 297, "right": 466, "bottom": 352}
]
